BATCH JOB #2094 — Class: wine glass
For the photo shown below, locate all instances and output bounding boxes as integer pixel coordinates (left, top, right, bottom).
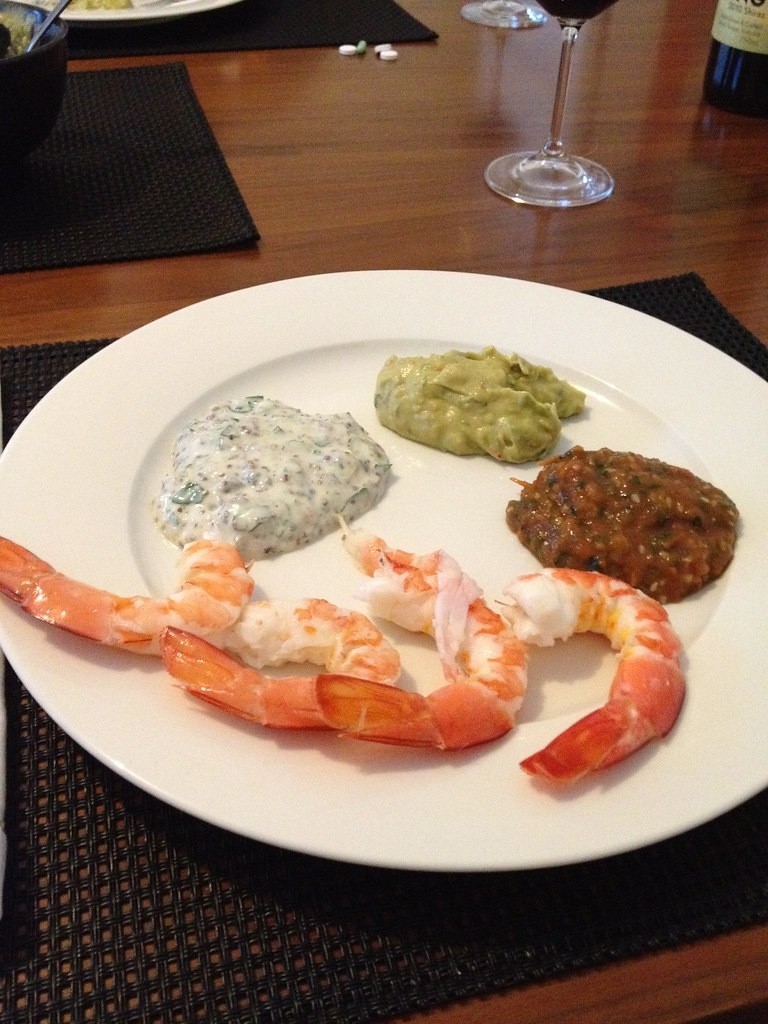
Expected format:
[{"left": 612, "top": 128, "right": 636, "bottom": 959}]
[
  {"left": 484, "top": 0, "right": 618, "bottom": 207},
  {"left": 460, "top": 1, "right": 547, "bottom": 27}
]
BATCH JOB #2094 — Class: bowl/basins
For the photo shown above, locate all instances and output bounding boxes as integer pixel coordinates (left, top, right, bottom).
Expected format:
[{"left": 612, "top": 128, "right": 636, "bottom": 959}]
[{"left": 0, "top": 0, "right": 69, "bottom": 168}]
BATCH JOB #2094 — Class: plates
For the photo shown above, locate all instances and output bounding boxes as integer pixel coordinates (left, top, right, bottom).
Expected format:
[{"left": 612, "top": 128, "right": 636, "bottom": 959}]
[
  {"left": 2, "top": 272, "right": 767, "bottom": 870},
  {"left": 29, "top": 0, "right": 244, "bottom": 23}
]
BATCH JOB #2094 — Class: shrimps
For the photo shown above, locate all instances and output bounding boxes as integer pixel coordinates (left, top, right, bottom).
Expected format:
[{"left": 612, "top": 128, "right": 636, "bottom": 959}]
[
  {"left": 158, "top": 595, "right": 402, "bottom": 732},
  {"left": 316, "top": 533, "right": 530, "bottom": 750},
  {"left": 0, "top": 534, "right": 254, "bottom": 654},
  {"left": 492, "top": 567, "right": 686, "bottom": 788}
]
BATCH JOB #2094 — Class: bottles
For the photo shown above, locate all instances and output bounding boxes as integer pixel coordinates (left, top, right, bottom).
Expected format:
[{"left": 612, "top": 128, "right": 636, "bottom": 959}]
[{"left": 704, "top": 0, "right": 768, "bottom": 118}]
